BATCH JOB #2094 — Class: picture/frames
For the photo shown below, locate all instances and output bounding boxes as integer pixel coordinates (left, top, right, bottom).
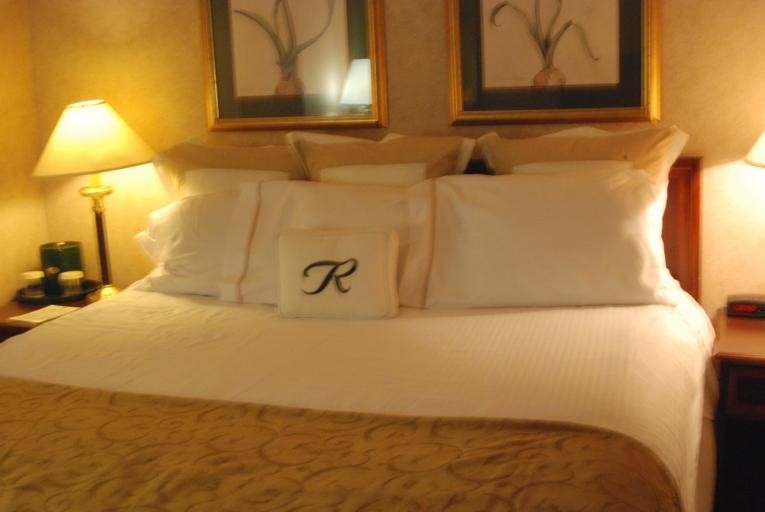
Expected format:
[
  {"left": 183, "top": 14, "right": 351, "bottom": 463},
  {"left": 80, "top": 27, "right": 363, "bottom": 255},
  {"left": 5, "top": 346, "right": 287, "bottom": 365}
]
[
  {"left": 444, "top": 0, "right": 663, "bottom": 125},
  {"left": 198, "top": 0, "right": 389, "bottom": 131}
]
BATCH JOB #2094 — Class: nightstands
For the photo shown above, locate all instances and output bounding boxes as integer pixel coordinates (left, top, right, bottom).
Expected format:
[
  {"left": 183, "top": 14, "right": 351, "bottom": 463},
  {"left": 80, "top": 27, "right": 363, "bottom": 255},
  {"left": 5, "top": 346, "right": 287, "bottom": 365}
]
[
  {"left": 714, "top": 307, "right": 765, "bottom": 421},
  {"left": 0, "top": 284, "right": 125, "bottom": 335}
]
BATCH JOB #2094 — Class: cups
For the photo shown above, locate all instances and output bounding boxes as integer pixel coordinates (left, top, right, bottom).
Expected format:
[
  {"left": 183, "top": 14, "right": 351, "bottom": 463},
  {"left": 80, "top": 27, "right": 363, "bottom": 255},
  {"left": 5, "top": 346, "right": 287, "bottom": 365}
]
[
  {"left": 21, "top": 270, "right": 45, "bottom": 298},
  {"left": 58, "top": 270, "right": 83, "bottom": 296}
]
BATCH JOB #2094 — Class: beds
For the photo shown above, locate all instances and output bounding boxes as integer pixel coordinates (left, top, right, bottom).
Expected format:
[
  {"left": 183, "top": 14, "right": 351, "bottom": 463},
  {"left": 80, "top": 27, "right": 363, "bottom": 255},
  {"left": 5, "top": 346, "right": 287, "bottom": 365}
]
[{"left": 0, "top": 159, "right": 715, "bottom": 512}]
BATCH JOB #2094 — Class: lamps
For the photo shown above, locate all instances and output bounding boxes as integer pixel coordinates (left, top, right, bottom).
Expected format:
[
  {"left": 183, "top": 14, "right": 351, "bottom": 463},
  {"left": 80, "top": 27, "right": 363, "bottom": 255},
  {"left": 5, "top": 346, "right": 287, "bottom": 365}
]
[
  {"left": 744, "top": 128, "right": 765, "bottom": 168},
  {"left": 32, "top": 99, "right": 162, "bottom": 300}
]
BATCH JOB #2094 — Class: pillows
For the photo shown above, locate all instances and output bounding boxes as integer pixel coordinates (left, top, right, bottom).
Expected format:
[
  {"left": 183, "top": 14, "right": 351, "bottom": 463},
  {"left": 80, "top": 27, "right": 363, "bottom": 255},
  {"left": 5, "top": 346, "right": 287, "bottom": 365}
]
[{"left": 135, "top": 124, "right": 690, "bottom": 318}]
